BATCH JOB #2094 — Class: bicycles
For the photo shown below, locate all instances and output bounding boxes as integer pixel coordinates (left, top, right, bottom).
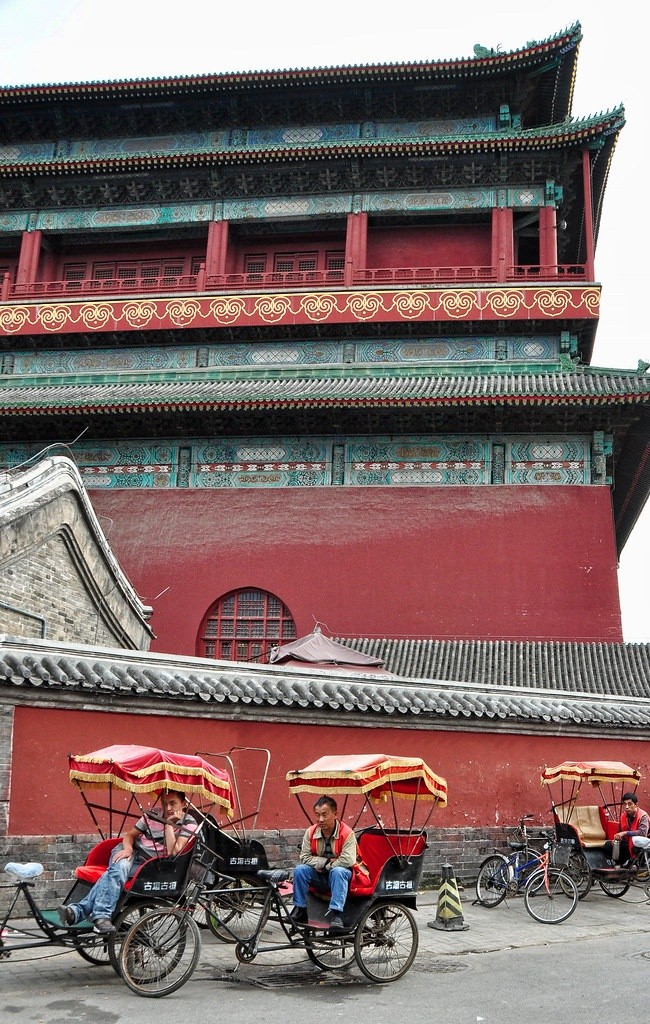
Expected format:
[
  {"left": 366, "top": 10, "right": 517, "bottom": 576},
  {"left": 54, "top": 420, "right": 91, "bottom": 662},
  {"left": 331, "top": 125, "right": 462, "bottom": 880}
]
[{"left": 472, "top": 813, "right": 578, "bottom": 924}]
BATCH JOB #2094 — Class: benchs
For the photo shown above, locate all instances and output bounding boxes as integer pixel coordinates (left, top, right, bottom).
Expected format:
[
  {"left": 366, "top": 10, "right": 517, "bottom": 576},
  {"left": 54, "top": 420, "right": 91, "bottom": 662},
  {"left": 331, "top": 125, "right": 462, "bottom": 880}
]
[
  {"left": 552, "top": 804, "right": 621, "bottom": 849},
  {"left": 304, "top": 827, "right": 428, "bottom": 901},
  {"left": 179, "top": 808, "right": 269, "bottom": 873},
  {"left": 73, "top": 825, "right": 198, "bottom": 902}
]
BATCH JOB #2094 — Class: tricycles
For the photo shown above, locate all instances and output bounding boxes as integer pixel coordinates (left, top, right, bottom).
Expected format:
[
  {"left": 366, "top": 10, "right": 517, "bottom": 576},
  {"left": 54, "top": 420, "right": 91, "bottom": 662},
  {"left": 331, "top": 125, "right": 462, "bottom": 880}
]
[
  {"left": 0, "top": 743, "right": 235, "bottom": 998},
  {"left": 228, "top": 754, "right": 449, "bottom": 982},
  {"left": 190, "top": 744, "right": 294, "bottom": 943},
  {"left": 539, "top": 761, "right": 650, "bottom": 904}
]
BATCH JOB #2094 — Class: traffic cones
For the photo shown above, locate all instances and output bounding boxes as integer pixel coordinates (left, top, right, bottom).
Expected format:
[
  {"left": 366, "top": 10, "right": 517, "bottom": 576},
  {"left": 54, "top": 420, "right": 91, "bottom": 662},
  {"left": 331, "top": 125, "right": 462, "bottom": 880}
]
[{"left": 428, "top": 863, "right": 470, "bottom": 933}]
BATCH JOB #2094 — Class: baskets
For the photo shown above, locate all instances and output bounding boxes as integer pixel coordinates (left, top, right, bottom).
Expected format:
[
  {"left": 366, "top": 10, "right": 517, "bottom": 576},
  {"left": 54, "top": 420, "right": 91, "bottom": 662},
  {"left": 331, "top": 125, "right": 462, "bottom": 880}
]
[
  {"left": 507, "top": 826, "right": 525, "bottom": 843},
  {"left": 551, "top": 842, "right": 572, "bottom": 868}
]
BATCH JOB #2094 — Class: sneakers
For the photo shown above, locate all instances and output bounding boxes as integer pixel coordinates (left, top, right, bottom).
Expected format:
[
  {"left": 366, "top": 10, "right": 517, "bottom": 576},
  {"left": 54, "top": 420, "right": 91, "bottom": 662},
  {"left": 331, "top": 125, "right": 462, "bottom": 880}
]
[
  {"left": 281, "top": 905, "right": 309, "bottom": 923},
  {"left": 328, "top": 910, "right": 344, "bottom": 928},
  {"left": 92, "top": 918, "right": 116, "bottom": 933},
  {"left": 57, "top": 904, "right": 75, "bottom": 926}
]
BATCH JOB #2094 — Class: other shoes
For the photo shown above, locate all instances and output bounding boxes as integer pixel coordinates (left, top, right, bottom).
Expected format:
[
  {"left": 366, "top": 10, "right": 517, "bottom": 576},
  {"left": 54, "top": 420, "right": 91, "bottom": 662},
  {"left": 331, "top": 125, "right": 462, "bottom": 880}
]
[
  {"left": 613, "top": 859, "right": 634, "bottom": 869},
  {"left": 597, "top": 858, "right": 616, "bottom": 868}
]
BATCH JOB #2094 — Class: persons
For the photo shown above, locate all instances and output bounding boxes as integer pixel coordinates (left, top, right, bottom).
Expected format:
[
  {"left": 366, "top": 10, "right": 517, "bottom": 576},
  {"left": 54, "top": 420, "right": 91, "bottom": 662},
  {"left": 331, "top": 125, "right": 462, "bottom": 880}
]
[
  {"left": 277, "top": 796, "right": 372, "bottom": 929},
  {"left": 58, "top": 788, "right": 198, "bottom": 933},
  {"left": 604, "top": 792, "right": 650, "bottom": 868}
]
